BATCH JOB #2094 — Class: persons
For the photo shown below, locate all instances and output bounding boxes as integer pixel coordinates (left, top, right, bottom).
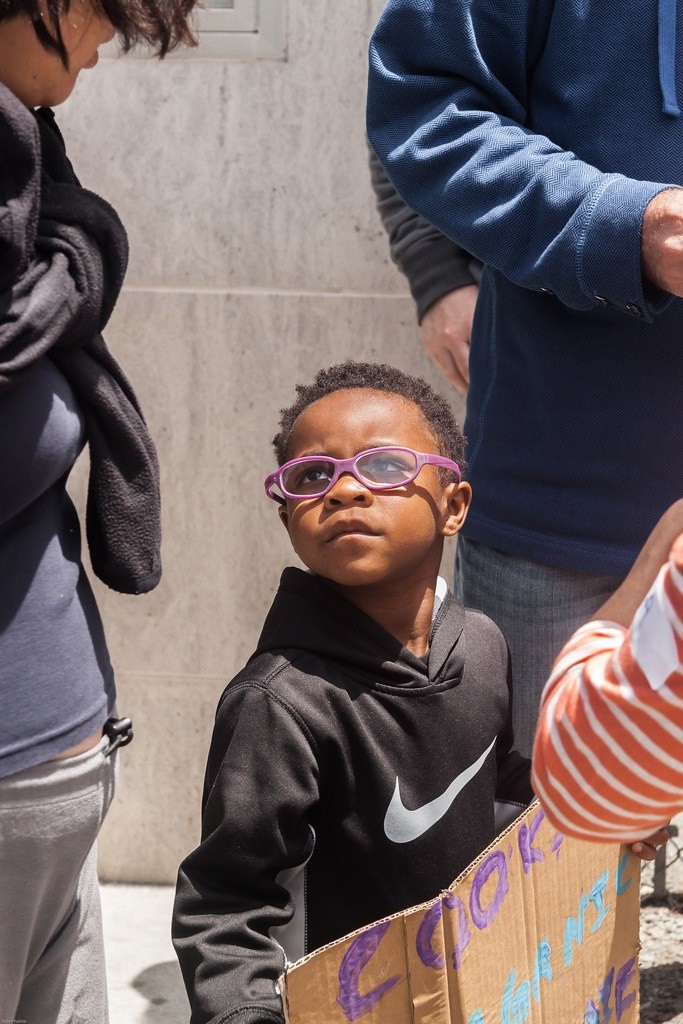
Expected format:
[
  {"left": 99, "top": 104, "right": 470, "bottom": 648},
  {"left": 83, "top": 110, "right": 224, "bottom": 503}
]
[
  {"left": 0, "top": 0, "right": 198, "bottom": 1024},
  {"left": 175, "top": 364, "right": 668, "bottom": 1024},
  {"left": 365, "top": 0, "right": 683, "bottom": 846}
]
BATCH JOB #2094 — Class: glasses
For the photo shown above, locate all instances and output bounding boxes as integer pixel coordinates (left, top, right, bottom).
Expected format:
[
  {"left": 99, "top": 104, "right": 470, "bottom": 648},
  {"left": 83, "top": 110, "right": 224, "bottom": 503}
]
[{"left": 263, "top": 446, "right": 461, "bottom": 506}]
